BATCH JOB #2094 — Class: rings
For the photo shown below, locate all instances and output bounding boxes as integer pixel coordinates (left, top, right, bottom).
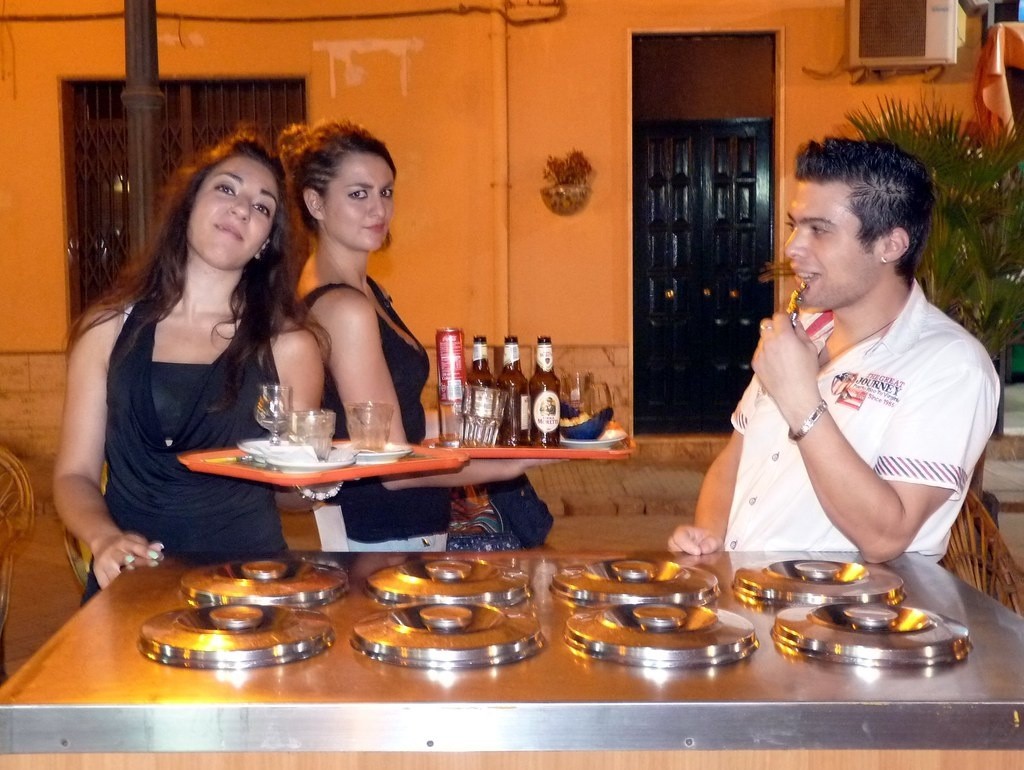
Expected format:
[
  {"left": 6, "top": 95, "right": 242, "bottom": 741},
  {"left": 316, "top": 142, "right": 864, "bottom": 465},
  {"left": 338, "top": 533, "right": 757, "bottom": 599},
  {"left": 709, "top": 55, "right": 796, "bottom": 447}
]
[{"left": 759, "top": 324, "right": 773, "bottom": 335}]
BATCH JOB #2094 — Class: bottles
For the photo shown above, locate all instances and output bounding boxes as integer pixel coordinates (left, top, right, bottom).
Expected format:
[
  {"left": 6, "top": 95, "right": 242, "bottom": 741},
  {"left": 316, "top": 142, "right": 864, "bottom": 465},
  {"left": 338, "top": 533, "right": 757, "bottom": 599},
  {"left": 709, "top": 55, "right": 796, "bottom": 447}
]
[
  {"left": 529, "top": 335, "right": 560, "bottom": 448},
  {"left": 496, "top": 336, "right": 529, "bottom": 448},
  {"left": 466, "top": 335, "right": 496, "bottom": 387}
]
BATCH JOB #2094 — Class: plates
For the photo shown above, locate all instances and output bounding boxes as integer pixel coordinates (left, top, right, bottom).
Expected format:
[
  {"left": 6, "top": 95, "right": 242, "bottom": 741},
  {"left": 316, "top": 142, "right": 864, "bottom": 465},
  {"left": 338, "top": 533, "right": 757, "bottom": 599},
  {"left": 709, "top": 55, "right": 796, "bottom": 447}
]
[
  {"left": 265, "top": 446, "right": 355, "bottom": 473},
  {"left": 236, "top": 438, "right": 289, "bottom": 464},
  {"left": 559, "top": 426, "right": 628, "bottom": 450},
  {"left": 336, "top": 442, "right": 413, "bottom": 465}
]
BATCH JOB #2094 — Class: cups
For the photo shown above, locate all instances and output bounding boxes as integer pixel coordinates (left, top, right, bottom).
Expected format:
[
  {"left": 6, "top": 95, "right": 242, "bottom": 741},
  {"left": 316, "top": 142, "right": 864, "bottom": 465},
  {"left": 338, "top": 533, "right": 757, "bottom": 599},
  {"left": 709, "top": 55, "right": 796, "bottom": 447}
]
[
  {"left": 344, "top": 403, "right": 394, "bottom": 453},
  {"left": 561, "top": 371, "right": 612, "bottom": 417},
  {"left": 290, "top": 408, "right": 339, "bottom": 462},
  {"left": 438, "top": 386, "right": 466, "bottom": 447},
  {"left": 462, "top": 383, "right": 508, "bottom": 449}
]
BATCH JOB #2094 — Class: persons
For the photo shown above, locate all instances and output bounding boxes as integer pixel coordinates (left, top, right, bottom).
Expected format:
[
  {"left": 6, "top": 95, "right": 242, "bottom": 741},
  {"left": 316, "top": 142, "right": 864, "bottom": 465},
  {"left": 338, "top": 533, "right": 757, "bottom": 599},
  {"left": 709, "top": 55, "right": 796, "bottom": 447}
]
[
  {"left": 279, "top": 117, "right": 568, "bottom": 552},
  {"left": 667, "top": 136, "right": 1000, "bottom": 564},
  {"left": 53, "top": 135, "right": 360, "bottom": 608}
]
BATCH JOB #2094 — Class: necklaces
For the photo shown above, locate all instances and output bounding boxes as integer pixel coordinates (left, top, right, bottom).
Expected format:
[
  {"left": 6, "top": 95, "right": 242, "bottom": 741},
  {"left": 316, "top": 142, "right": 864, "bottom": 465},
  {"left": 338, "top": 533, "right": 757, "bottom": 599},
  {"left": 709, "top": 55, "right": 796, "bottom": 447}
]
[{"left": 820, "top": 319, "right": 894, "bottom": 368}]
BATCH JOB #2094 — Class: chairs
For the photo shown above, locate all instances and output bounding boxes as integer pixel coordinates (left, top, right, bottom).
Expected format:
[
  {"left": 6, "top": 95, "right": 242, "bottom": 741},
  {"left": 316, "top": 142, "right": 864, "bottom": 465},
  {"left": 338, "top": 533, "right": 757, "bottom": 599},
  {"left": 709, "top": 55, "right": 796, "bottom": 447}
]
[
  {"left": 937, "top": 493, "right": 1023, "bottom": 615},
  {"left": 0, "top": 445, "right": 36, "bottom": 687}
]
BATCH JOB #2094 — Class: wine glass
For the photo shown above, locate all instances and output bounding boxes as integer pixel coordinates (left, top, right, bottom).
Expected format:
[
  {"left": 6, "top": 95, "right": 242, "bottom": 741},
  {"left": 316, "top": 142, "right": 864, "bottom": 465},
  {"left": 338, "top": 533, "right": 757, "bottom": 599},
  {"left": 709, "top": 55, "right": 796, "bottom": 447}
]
[{"left": 254, "top": 384, "right": 292, "bottom": 456}]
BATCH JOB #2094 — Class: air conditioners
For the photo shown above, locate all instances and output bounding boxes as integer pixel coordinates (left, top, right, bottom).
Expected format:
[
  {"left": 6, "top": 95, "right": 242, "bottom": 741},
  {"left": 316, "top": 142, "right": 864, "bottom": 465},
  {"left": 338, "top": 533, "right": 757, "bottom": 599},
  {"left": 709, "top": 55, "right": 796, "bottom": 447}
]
[{"left": 849, "top": 0, "right": 958, "bottom": 71}]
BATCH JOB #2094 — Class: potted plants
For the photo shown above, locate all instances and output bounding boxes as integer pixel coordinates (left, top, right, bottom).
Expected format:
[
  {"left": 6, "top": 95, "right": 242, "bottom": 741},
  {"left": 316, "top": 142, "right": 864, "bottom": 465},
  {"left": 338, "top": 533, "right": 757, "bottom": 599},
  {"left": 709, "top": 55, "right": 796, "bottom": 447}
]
[{"left": 539, "top": 146, "right": 593, "bottom": 216}]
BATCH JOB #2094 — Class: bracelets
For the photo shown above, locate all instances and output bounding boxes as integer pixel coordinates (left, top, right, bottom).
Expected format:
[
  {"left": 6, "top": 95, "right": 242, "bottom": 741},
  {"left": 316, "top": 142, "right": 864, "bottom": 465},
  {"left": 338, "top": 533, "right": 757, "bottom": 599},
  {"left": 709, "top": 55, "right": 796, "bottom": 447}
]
[
  {"left": 787, "top": 399, "right": 827, "bottom": 441},
  {"left": 293, "top": 481, "right": 343, "bottom": 503}
]
